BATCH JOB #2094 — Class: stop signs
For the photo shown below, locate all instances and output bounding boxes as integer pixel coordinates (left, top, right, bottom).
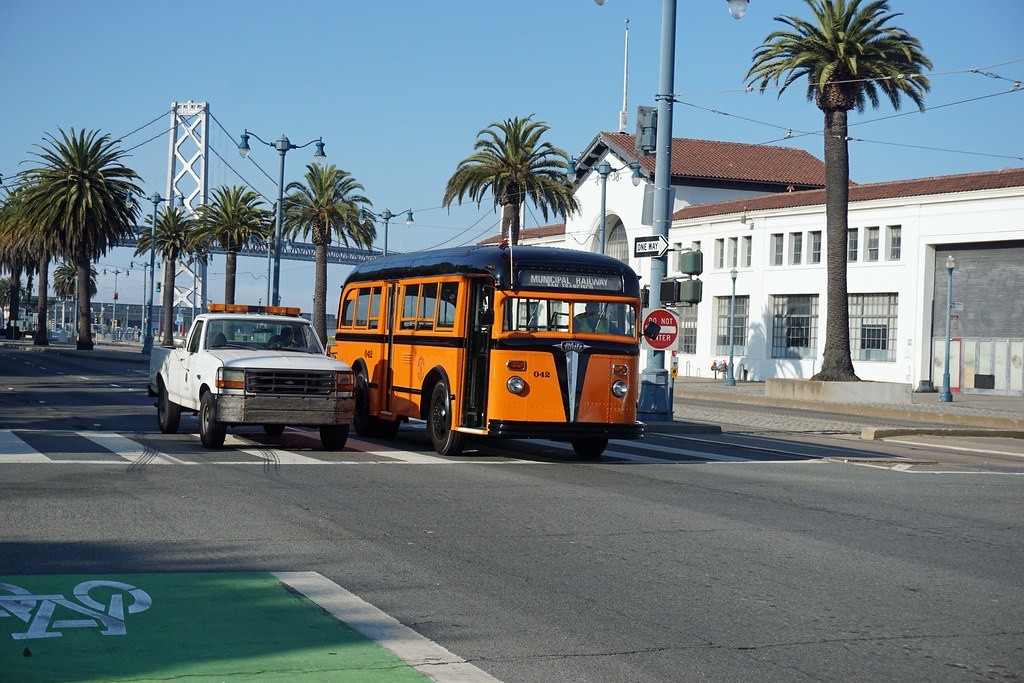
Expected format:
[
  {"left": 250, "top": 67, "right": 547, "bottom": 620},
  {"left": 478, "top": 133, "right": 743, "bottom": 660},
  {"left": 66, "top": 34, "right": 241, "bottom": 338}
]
[{"left": 642, "top": 309, "right": 679, "bottom": 349}]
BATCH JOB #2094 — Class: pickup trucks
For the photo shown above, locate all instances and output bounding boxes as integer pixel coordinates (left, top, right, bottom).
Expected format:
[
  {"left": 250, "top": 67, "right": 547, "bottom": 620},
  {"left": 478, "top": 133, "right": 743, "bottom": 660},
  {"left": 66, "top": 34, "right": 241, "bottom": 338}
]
[{"left": 146, "top": 304, "right": 362, "bottom": 451}]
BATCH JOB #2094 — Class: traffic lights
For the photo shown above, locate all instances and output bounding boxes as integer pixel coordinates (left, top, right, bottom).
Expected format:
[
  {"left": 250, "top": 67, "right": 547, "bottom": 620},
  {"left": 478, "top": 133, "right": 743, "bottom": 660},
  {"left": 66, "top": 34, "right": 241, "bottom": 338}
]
[{"left": 156, "top": 282, "right": 161, "bottom": 292}]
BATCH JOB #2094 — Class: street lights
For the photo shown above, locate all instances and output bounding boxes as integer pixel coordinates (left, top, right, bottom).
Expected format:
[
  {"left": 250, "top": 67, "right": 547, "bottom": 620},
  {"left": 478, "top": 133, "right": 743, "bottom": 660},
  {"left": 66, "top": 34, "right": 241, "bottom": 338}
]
[
  {"left": 65, "top": 272, "right": 79, "bottom": 333},
  {"left": 725, "top": 268, "right": 740, "bottom": 388},
  {"left": 126, "top": 190, "right": 184, "bottom": 354},
  {"left": 358, "top": 206, "right": 416, "bottom": 257},
  {"left": 102, "top": 267, "right": 131, "bottom": 341},
  {"left": 128, "top": 259, "right": 161, "bottom": 342},
  {"left": 237, "top": 130, "right": 328, "bottom": 306},
  {"left": 937, "top": 256, "right": 958, "bottom": 401},
  {"left": 566, "top": 155, "right": 643, "bottom": 256},
  {"left": 265, "top": 235, "right": 273, "bottom": 308}
]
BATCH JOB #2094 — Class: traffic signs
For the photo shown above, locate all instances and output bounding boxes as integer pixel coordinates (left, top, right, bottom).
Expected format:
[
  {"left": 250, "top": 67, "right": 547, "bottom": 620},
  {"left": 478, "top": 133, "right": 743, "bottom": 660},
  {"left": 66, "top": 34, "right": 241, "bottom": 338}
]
[{"left": 633, "top": 234, "right": 669, "bottom": 258}]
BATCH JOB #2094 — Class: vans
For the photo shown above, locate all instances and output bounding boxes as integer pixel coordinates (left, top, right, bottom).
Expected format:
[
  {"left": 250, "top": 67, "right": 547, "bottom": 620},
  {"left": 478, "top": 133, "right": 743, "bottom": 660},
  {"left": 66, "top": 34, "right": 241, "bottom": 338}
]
[{"left": 327, "top": 242, "right": 662, "bottom": 459}]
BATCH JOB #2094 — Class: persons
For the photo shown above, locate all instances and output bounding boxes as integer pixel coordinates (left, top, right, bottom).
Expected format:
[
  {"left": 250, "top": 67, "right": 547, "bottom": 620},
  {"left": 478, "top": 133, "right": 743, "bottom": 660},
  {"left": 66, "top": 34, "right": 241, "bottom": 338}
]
[
  {"left": 573, "top": 302, "right": 624, "bottom": 334},
  {"left": 268, "top": 327, "right": 303, "bottom": 349}
]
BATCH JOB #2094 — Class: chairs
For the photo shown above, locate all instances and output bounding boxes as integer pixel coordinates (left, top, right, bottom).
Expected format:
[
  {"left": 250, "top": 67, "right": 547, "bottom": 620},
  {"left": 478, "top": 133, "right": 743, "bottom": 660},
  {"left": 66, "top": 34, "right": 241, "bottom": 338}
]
[
  {"left": 206, "top": 331, "right": 228, "bottom": 347},
  {"left": 267, "top": 335, "right": 281, "bottom": 347}
]
